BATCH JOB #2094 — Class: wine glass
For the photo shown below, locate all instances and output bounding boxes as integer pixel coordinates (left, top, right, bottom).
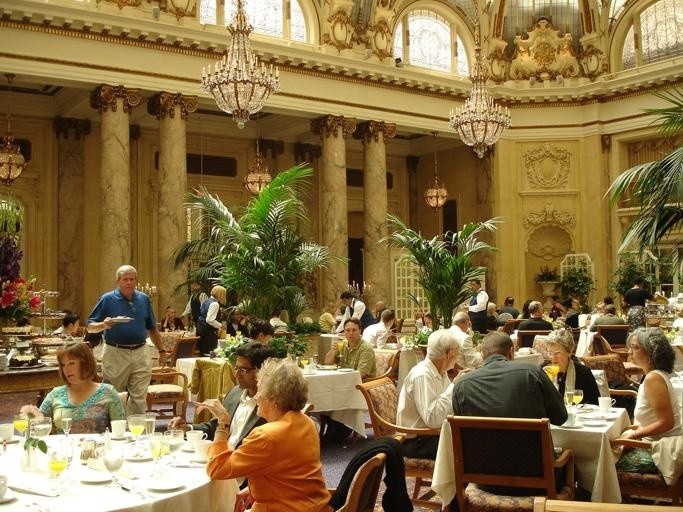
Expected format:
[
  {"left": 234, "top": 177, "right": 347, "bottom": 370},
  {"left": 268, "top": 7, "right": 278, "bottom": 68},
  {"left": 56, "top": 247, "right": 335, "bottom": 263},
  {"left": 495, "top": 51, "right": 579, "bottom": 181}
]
[
  {"left": 126, "top": 414, "right": 145, "bottom": 445},
  {"left": 336, "top": 343, "right": 344, "bottom": 357},
  {"left": 59, "top": 407, "right": 73, "bottom": 438},
  {"left": 573, "top": 389, "right": 583, "bottom": 409},
  {"left": 673, "top": 368, "right": 683, "bottom": 382},
  {"left": 171, "top": 325, "right": 174, "bottom": 331},
  {"left": 12, "top": 415, "right": 28, "bottom": 442}
]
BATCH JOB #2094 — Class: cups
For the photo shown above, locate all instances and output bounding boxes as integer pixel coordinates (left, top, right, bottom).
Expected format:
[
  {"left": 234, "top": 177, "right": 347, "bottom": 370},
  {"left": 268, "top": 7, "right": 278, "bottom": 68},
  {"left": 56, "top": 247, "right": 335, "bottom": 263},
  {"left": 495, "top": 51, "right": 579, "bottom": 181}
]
[
  {"left": 32, "top": 417, "right": 51, "bottom": 436},
  {"left": 109, "top": 420, "right": 126, "bottom": 437},
  {"left": 164, "top": 329, "right": 168, "bottom": 333},
  {"left": 235, "top": 330, "right": 240, "bottom": 336},
  {"left": 193, "top": 439, "right": 212, "bottom": 458},
  {"left": 597, "top": 396, "right": 615, "bottom": 412},
  {"left": 163, "top": 430, "right": 184, "bottom": 453},
  {"left": 144, "top": 413, "right": 154, "bottom": 436},
  {"left": 0, "top": 353, "right": 7, "bottom": 370},
  {"left": 563, "top": 392, "right": 573, "bottom": 407},
  {"left": 186, "top": 430, "right": 207, "bottom": 447}
]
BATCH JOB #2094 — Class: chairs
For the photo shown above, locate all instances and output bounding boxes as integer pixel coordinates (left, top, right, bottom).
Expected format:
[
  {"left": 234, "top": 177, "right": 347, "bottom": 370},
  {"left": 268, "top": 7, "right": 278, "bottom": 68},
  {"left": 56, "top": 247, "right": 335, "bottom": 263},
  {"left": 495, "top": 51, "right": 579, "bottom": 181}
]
[{"left": 0, "top": 321, "right": 680, "bottom": 511}]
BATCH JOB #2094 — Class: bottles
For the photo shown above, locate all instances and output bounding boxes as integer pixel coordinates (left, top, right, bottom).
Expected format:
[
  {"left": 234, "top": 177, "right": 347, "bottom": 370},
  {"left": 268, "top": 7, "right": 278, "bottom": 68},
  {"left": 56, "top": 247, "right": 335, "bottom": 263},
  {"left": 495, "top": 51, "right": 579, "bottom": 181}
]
[
  {"left": 295, "top": 351, "right": 318, "bottom": 374},
  {"left": 219, "top": 320, "right": 226, "bottom": 339},
  {"left": 102, "top": 426, "right": 111, "bottom": 451}
]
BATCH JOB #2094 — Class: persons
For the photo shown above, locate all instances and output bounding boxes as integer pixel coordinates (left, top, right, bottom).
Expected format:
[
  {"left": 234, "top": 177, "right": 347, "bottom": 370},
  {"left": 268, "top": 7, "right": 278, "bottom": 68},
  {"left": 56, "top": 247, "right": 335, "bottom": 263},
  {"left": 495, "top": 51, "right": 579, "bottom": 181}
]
[
  {"left": 179, "top": 284, "right": 286, "bottom": 357},
  {"left": 195, "top": 358, "right": 333, "bottom": 512},
  {"left": 166, "top": 343, "right": 275, "bottom": 491},
  {"left": 318, "top": 291, "right": 403, "bottom": 450},
  {"left": 61, "top": 310, "right": 106, "bottom": 360},
  {"left": 85, "top": 265, "right": 169, "bottom": 422},
  {"left": 396, "top": 286, "right": 682, "bottom": 512},
  {"left": 38, "top": 342, "right": 128, "bottom": 433},
  {"left": 459, "top": 279, "right": 489, "bottom": 334}
]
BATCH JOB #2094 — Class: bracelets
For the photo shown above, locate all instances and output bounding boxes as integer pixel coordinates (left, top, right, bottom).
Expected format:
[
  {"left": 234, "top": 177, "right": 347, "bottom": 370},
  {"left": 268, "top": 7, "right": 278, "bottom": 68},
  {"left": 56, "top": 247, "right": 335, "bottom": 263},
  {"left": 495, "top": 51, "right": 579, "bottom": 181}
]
[
  {"left": 216, "top": 430, "right": 229, "bottom": 436},
  {"left": 158, "top": 349, "right": 165, "bottom": 353}
]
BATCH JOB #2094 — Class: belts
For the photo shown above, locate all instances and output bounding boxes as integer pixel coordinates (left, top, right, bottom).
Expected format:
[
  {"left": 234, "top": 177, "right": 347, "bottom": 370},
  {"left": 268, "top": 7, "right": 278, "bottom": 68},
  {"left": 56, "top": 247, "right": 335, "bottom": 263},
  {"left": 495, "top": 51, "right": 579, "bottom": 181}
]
[{"left": 106, "top": 341, "right": 145, "bottom": 350}]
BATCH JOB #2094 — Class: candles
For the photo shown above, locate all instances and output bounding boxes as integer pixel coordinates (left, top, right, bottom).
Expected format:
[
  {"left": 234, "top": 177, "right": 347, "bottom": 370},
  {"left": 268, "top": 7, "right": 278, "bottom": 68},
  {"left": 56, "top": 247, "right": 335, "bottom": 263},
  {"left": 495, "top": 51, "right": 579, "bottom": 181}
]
[{"left": 345, "top": 278, "right": 372, "bottom": 293}]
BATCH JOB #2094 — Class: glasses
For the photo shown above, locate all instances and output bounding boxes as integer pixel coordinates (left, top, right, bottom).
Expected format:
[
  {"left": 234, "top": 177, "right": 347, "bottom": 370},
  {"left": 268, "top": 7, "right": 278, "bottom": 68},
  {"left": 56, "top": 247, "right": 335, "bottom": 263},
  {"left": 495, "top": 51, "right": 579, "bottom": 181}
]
[{"left": 234, "top": 365, "right": 254, "bottom": 374}]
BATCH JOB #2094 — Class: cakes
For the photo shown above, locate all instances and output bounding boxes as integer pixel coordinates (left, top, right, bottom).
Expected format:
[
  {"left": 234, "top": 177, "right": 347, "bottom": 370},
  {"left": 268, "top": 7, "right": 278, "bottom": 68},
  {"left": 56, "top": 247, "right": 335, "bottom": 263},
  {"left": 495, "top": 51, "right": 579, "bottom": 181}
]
[
  {"left": 40, "top": 355, "right": 58, "bottom": 363},
  {"left": 9, "top": 336, "right": 30, "bottom": 348},
  {"left": 2, "top": 324, "right": 33, "bottom": 334},
  {"left": 10, "top": 355, "right": 38, "bottom": 366},
  {"left": 32, "top": 337, "right": 63, "bottom": 345},
  {"left": 0, "top": 353, "right": 9, "bottom": 372}
]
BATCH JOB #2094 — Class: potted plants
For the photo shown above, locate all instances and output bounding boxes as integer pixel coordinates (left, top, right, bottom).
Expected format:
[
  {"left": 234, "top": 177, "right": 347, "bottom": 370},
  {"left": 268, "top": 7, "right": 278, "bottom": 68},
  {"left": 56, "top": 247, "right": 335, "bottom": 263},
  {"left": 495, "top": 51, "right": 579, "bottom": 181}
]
[{"left": 531, "top": 263, "right": 559, "bottom": 312}]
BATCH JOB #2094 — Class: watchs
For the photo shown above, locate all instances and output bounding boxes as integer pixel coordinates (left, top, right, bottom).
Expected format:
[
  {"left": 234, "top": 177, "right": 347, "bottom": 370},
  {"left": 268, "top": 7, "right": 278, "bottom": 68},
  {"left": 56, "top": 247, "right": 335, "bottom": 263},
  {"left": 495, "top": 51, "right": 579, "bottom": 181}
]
[{"left": 216, "top": 422, "right": 229, "bottom": 428}]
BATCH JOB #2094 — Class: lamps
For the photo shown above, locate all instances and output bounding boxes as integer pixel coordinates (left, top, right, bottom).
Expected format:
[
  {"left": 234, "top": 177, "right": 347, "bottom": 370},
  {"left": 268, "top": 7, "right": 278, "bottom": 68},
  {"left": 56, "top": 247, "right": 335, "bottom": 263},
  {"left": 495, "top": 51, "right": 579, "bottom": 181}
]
[
  {"left": 445, "top": 0, "right": 511, "bottom": 158},
  {"left": 421, "top": 130, "right": 450, "bottom": 212},
  {"left": 197, "top": 0, "right": 279, "bottom": 131},
  {"left": 0, "top": 96, "right": 28, "bottom": 187},
  {"left": 243, "top": 107, "right": 275, "bottom": 197}
]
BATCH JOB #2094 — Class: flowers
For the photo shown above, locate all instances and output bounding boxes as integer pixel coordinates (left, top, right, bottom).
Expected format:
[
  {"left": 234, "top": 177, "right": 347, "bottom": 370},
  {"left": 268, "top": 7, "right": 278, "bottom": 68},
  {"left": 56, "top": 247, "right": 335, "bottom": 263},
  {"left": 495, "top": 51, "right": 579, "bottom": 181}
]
[{"left": 0, "top": 277, "right": 44, "bottom": 322}]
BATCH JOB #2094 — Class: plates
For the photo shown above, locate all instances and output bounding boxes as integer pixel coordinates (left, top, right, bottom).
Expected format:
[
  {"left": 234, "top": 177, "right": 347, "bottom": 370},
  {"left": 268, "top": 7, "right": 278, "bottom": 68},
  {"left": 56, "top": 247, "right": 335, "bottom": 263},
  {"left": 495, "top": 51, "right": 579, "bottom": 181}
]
[
  {"left": 0, "top": 433, "right": 207, "bottom": 505},
  {"left": 110, "top": 317, "right": 133, "bottom": 324},
  {"left": 338, "top": 368, "right": 352, "bottom": 371},
  {"left": 560, "top": 404, "right": 619, "bottom": 429},
  {"left": 317, "top": 364, "right": 337, "bottom": 369},
  {"left": 39, "top": 355, "right": 57, "bottom": 367}
]
